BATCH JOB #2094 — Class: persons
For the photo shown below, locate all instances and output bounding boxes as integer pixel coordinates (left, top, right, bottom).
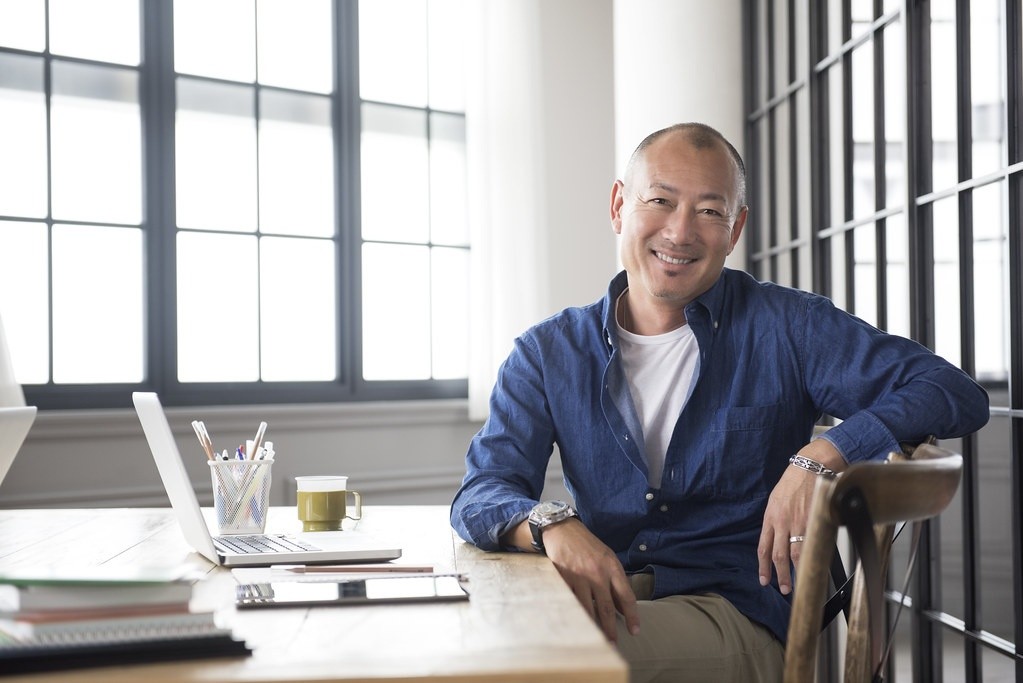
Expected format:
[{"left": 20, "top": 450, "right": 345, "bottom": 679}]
[{"left": 446, "top": 119, "right": 989, "bottom": 682}]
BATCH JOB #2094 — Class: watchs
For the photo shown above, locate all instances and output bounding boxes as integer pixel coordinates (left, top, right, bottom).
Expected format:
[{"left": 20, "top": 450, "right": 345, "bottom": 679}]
[{"left": 526, "top": 500, "right": 585, "bottom": 556}]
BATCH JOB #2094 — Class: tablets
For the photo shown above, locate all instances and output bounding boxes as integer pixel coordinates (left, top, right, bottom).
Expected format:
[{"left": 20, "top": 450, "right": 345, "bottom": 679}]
[{"left": 273, "top": 576, "right": 470, "bottom": 606}]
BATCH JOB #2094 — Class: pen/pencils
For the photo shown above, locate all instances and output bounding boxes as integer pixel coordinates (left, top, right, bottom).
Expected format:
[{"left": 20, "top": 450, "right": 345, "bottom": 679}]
[
  {"left": 191, "top": 419, "right": 276, "bottom": 532},
  {"left": 284, "top": 567, "right": 433, "bottom": 574}
]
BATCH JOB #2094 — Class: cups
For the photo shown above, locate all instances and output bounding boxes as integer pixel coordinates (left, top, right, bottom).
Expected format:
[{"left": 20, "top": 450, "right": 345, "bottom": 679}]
[{"left": 295, "top": 476, "right": 362, "bottom": 532}]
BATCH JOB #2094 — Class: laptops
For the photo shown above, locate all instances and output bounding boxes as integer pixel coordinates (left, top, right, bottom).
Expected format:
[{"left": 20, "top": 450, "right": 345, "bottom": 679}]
[{"left": 132, "top": 391, "right": 402, "bottom": 568}]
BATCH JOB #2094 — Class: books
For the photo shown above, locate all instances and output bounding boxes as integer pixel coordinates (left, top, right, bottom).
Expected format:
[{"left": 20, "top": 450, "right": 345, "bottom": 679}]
[{"left": 0, "top": 573, "right": 252, "bottom": 677}]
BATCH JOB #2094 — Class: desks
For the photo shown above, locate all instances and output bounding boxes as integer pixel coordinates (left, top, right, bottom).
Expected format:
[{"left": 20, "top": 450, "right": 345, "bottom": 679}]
[{"left": 0, "top": 505, "right": 629, "bottom": 683}]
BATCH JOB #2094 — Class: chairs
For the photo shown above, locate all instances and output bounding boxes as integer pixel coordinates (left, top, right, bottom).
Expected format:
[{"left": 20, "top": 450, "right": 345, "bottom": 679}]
[{"left": 779, "top": 420, "right": 965, "bottom": 683}]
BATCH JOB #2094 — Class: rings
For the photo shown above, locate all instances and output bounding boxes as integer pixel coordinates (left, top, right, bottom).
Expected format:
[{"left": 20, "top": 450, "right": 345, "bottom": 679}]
[{"left": 787, "top": 535, "right": 804, "bottom": 543}]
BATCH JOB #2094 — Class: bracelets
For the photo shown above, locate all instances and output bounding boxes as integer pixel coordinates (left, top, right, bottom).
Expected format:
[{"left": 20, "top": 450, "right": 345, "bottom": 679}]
[{"left": 787, "top": 453, "right": 841, "bottom": 479}]
